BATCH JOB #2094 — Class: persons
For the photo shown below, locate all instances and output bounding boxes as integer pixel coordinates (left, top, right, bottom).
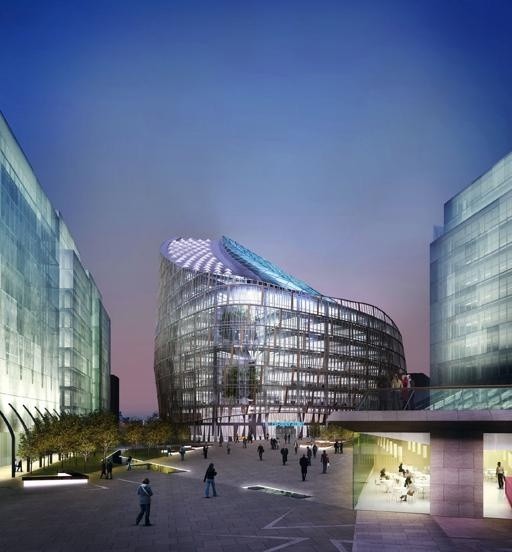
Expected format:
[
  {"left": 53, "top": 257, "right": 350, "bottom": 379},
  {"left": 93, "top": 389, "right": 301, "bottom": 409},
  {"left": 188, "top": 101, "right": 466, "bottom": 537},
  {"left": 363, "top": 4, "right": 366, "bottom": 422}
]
[
  {"left": 234, "top": 433, "right": 240, "bottom": 447},
  {"left": 496, "top": 462, "right": 505, "bottom": 489},
  {"left": 218, "top": 433, "right": 224, "bottom": 448},
  {"left": 299, "top": 453, "right": 309, "bottom": 482},
  {"left": 334, "top": 439, "right": 344, "bottom": 455},
  {"left": 396, "top": 463, "right": 416, "bottom": 502},
  {"left": 306, "top": 447, "right": 311, "bottom": 466},
  {"left": 321, "top": 450, "right": 329, "bottom": 475},
  {"left": 227, "top": 443, "right": 231, "bottom": 455},
  {"left": 99, "top": 460, "right": 112, "bottom": 480},
  {"left": 257, "top": 443, "right": 264, "bottom": 461},
  {"left": 203, "top": 462, "right": 217, "bottom": 498},
  {"left": 242, "top": 438, "right": 247, "bottom": 449},
  {"left": 136, "top": 478, "right": 153, "bottom": 526},
  {"left": 293, "top": 439, "right": 299, "bottom": 455},
  {"left": 284, "top": 433, "right": 291, "bottom": 445},
  {"left": 392, "top": 373, "right": 416, "bottom": 411},
  {"left": 203, "top": 445, "right": 209, "bottom": 459},
  {"left": 271, "top": 437, "right": 281, "bottom": 451},
  {"left": 267, "top": 433, "right": 269, "bottom": 440},
  {"left": 280, "top": 444, "right": 289, "bottom": 466},
  {"left": 17, "top": 459, "right": 22, "bottom": 473},
  {"left": 312, "top": 443, "right": 317, "bottom": 459},
  {"left": 126, "top": 456, "right": 133, "bottom": 470},
  {"left": 179, "top": 444, "right": 185, "bottom": 462},
  {"left": 247, "top": 431, "right": 253, "bottom": 443},
  {"left": 380, "top": 468, "right": 386, "bottom": 478}
]
